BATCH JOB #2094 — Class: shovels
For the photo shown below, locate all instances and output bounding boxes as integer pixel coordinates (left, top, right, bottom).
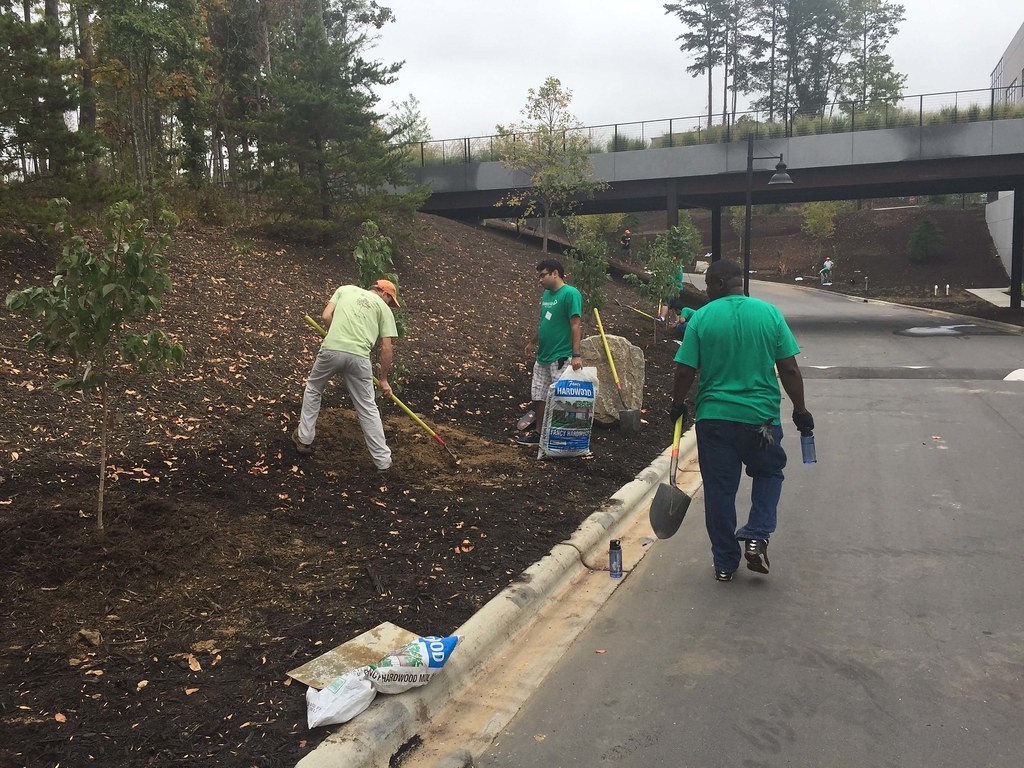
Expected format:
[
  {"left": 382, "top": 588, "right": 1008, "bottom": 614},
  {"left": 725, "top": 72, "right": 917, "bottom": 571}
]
[
  {"left": 594, "top": 307, "right": 640, "bottom": 436},
  {"left": 305, "top": 314, "right": 461, "bottom": 471},
  {"left": 650, "top": 413, "right": 692, "bottom": 539}
]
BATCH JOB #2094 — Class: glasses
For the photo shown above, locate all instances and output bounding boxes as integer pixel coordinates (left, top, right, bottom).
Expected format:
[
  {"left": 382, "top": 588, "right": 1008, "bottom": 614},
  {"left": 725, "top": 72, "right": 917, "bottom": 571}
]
[{"left": 539, "top": 273, "right": 552, "bottom": 279}]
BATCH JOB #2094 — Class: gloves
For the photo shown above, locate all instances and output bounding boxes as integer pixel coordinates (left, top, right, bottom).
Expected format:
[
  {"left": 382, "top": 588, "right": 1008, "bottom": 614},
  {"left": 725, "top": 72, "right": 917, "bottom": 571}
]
[
  {"left": 755, "top": 417, "right": 775, "bottom": 448},
  {"left": 669, "top": 406, "right": 688, "bottom": 427},
  {"left": 791, "top": 408, "right": 814, "bottom": 432}
]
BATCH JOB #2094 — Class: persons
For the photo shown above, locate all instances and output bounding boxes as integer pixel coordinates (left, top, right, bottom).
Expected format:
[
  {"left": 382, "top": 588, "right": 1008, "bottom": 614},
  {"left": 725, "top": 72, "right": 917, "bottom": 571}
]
[
  {"left": 621, "top": 230, "right": 631, "bottom": 266},
  {"left": 824, "top": 257, "right": 834, "bottom": 283},
  {"left": 293, "top": 280, "right": 400, "bottom": 479},
  {"left": 671, "top": 303, "right": 696, "bottom": 336},
  {"left": 671, "top": 259, "right": 815, "bottom": 581},
  {"left": 517, "top": 260, "right": 582, "bottom": 446},
  {"left": 820, "top": 266, "right": 832, "bottom": 285}
]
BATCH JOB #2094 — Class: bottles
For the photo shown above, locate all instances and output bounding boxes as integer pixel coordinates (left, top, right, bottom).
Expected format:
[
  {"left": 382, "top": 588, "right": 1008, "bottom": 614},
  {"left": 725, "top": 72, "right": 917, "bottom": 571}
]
[
  {"left": 799, "top": 430, "right": 817, "bottom": 464},
  {"left": 609, "top": 539, "right": 622, "bottom": 578}
]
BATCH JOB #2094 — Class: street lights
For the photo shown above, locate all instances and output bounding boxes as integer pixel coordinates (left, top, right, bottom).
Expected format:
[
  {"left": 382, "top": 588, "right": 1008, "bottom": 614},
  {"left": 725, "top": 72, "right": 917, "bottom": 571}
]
[{"left": 743, "top": 152, "right": 795, "bottom": 297}]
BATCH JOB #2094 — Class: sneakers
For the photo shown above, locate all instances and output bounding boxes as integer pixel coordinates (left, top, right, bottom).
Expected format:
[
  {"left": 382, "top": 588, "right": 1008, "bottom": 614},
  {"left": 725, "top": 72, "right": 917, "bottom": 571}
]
[
  {"left": 378, "top": 462, "right": 393, "bottom": 473},
  {"left": 715, "top": 570, "right": 734, "bottom": 581},
  {"left": 517, "top": 430, "right": 541, "bottom": 445},
  {"left": 292, "top": 429, "right": 312, "bottom": 454},
  {"left": 744, "top": 538, "right": 770, "bottom": 573}
]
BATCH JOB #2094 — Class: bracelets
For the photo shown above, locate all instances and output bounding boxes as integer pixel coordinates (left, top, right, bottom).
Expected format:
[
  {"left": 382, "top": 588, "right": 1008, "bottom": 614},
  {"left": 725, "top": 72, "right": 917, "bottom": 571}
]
[{"left": 572, "top": 353, "right": 581, "bottom": 357}]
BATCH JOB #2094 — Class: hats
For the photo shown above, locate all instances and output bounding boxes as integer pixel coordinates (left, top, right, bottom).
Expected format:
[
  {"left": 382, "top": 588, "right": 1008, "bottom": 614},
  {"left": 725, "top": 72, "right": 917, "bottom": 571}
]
[
  {"left": 625, "top": 230, "right": 630, "bottom": 234},
  {"left": 827, "top": 257, "right": 830, "bottom": 259},
  {"left": 371, "top": 280, "right": 401, "bottom": 308}
]
